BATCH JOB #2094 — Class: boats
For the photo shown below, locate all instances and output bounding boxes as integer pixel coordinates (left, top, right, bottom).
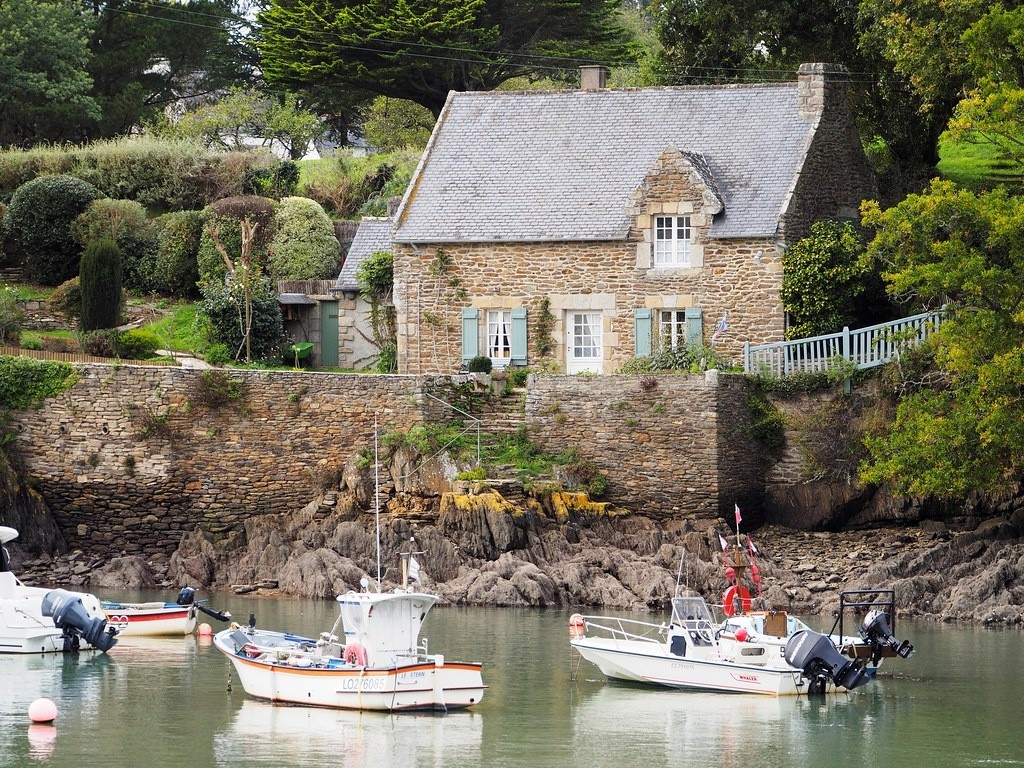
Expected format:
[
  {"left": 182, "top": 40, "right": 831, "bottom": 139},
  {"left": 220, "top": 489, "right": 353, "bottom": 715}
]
[
  {"left": 102, "top": 589, "right": 230, "bottom": 636},
  {"left": 566, "top": 501, "right": 916, "bottom": 700},
  {"left": 213, "top": 415, "right": 489, "bottom": 711},
  {"left": 0, "top": 525, "right": 120, "bottom": 654}
]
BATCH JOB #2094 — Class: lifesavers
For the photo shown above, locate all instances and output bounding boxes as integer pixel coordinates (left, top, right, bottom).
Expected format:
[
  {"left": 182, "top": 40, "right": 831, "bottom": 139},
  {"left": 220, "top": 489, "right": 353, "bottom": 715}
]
[
  {"left": 343, "top": 643, "right": 367, "bottom": 666},
  {"left": 722, "top": 584, "right": 752, "bottom": 616}
]
